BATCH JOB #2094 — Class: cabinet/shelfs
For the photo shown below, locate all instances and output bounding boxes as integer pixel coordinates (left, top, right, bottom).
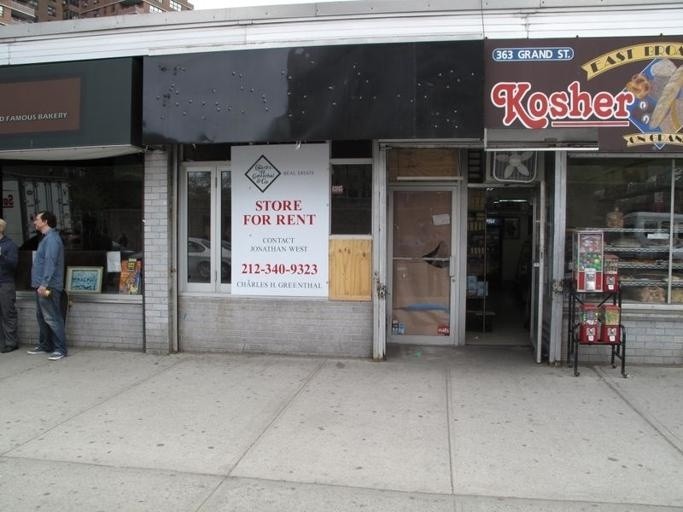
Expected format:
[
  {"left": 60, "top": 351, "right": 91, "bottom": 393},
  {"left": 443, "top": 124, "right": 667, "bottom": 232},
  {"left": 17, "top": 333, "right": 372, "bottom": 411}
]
[
  {"left": 604, "top": 203, "right": 683, "bottom": 304},
  {"left": 466, "top": 205, "right": 495, "bottom": 334},
  {"left": 568, "top": 278, "right": 627, "bottom": 379}
]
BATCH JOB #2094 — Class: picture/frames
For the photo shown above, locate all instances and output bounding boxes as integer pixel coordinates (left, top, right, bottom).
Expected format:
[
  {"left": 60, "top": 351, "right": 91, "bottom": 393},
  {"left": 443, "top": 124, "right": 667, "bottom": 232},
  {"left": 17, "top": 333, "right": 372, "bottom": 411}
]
[{"left": 65, "top": 266, "right": 102, "bottom": 293}]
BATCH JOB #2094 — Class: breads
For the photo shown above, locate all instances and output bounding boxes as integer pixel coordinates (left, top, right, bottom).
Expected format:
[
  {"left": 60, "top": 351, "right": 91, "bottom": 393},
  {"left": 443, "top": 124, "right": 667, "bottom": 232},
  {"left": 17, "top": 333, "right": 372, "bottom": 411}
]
[{"left": 626, "top": 61, "right": 683, "bottom": 132}]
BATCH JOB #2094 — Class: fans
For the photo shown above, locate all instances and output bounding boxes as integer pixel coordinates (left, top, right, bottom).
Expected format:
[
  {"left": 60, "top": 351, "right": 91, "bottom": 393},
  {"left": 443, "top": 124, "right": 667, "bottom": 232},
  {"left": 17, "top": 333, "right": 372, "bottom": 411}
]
[{"left": 489, "top": 147, "right": 539, "bottom": 185}]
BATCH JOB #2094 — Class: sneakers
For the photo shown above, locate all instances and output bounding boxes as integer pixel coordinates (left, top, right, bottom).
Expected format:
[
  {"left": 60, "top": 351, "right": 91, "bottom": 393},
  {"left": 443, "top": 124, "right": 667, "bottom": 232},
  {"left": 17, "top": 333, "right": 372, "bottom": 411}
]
[
  {"left": 27, "top": 346, "right": 48, "bottom": 354},
  {"left": 48, "top": 352, "right": 65, "bottom": 359},
  {"left": 2, "top": 346, "right": 18, "bottom": 352}
]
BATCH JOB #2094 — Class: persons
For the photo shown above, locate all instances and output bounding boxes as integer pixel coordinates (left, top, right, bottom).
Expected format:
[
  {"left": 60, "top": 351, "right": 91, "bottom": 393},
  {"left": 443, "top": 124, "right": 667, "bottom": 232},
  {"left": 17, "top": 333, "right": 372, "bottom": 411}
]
[
  {"left": 0, "top": 218, "right": 21, "bottom": 354},
  {"left": 25, "top": 210, "right": 68, "bottom": 361}
]
